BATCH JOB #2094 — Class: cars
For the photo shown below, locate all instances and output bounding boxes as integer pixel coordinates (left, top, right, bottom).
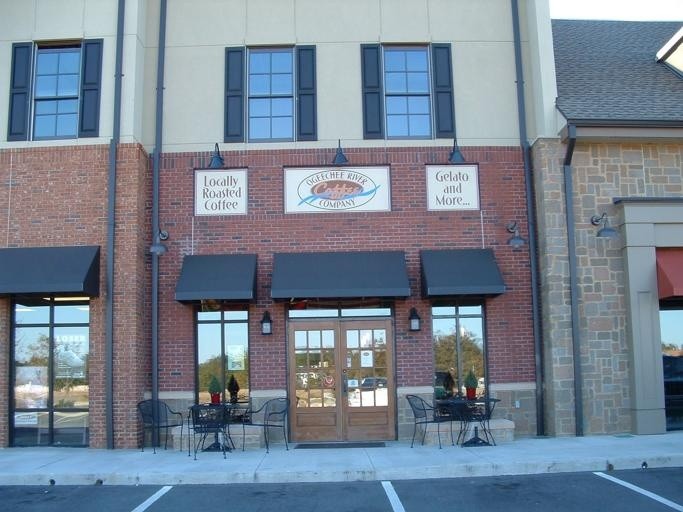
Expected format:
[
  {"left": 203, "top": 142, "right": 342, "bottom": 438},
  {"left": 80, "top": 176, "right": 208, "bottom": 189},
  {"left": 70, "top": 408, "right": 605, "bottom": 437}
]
[{"left": 359, "top": 378, "right": 387, "bottom": 391}]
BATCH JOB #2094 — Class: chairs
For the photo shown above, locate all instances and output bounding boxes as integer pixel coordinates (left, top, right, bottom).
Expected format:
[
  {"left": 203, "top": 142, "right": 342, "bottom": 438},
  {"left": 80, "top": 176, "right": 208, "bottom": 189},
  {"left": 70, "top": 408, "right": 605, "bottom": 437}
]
[
  {"left": 240, "top": 396, "right": 290, "bottom": 454},
  {"left": 189, "top": 403, "right": 229, "bottom": 459},
  {"left": 405, "top": 392, "right": 496, "bottom": 448},
  {"left": 136, "top": 399, "right": 182, "bottom": 454}
]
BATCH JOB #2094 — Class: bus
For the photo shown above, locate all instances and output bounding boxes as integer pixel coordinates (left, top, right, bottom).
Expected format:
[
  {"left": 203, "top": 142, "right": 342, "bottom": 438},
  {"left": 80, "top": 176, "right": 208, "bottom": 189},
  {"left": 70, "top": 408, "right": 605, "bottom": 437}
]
[{"left": 297, "top": 372, "right": 316, "bottom": 390}]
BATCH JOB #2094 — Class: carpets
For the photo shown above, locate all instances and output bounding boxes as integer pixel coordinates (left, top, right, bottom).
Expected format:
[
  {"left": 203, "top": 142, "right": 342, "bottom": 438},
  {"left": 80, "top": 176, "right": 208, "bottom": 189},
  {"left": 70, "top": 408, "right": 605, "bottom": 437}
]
[{"left": 292, "top": 441, "right": 385, "bottom": 449}]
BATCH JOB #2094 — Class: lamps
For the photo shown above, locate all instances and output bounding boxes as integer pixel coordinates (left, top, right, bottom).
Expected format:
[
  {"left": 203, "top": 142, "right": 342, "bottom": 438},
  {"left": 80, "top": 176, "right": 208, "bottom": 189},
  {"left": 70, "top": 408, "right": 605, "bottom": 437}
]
[
  {"left": 206, "top": 142, "right": 225, "bottom": 168},
  {"left": 149, "top": 228, "right": 168, "bottom": 256},
  {"left": 330, "top": 139, "right": 348, "bottom": 165},
  {"left": 408, "top": 306, "right": 421, "bottom": 331},
  {"left": 590, "top": 212, "right": 616, "bottom": 239},
  {"left": 259, "top": 310, "right": 272, "bottom": 336},
  {"left": 506, "top": 221, "right": 525, "bottom": 250},
  {"left": 448, "top": 139, "right": 465, "bottom": 164}
]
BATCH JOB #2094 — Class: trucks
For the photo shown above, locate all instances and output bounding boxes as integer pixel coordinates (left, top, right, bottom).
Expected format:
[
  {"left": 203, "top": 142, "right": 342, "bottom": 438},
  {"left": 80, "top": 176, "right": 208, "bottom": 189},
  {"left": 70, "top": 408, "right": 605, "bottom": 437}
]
[{"left": 334, "top": 380, "right": 358, "bottom": 391}]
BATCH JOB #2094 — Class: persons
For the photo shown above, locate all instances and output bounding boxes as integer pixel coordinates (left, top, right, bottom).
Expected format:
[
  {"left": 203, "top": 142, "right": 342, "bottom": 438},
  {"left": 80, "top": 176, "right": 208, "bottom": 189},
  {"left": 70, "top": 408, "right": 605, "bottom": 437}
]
[
  {"left": 227, "top": 375, "right": 240, "bottom": 403},
  {"left": 443, "top": 368, "right": 455, "bottom": 396}
]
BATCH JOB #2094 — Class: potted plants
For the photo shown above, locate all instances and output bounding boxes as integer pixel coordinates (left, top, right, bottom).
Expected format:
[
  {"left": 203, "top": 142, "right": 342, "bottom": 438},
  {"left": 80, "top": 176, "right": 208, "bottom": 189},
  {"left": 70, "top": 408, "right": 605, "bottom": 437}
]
[
  {"left": 207, "top": 374, "right": 221, "bottom": 406},
  {"left": 464, "top": 370, "right": 478, "bottom": 399}
]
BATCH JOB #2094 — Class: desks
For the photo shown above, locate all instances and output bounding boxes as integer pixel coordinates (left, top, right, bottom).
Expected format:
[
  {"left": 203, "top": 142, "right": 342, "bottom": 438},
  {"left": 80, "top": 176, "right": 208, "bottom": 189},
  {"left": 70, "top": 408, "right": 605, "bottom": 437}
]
[
  {"left": 435, "top": 397, "right": 500, "bottom": 447},
  {"left": 187, "top": 404, "right": 237, "bottom": 452}
]
